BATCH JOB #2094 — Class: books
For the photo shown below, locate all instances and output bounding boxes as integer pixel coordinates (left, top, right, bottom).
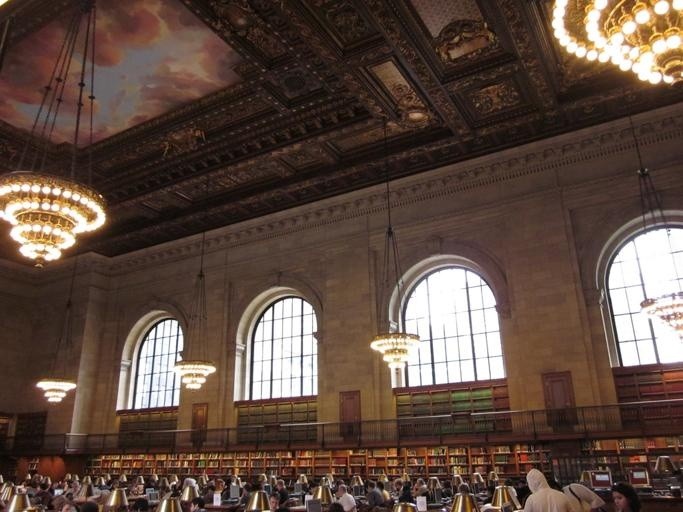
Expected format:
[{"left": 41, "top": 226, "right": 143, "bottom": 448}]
[{"left": 80, "top": 435, "right": 682, "bottom": 482}]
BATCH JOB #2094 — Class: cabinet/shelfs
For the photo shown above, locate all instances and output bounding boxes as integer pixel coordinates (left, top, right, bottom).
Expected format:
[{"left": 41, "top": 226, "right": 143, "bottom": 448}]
[{"left": 0, "top": 361, "right": 683, "bottom": 512}]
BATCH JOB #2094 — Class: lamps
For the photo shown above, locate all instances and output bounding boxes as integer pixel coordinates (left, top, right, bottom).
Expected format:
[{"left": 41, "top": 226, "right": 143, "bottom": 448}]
[
  {"left": 611, "top": 77, "right": 683, "bottom": 345},
  {"left": 33, "top": 234, "right": 77, "bottom": 408},
  {"left": 545, "top": 0, "right": 682, "bottom": 97},
  {"left": 367, "top": 113, "right": 424, "bottom": 375},
  {"left": 0, "top": 3, "right": 112, "bottom": 274},
  {"left": 162, "top": 165, "right": 216, "bottom": 395}
]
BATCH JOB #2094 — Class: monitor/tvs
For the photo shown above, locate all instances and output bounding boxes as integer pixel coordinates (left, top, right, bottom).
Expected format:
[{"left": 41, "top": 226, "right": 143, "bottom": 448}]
[
  {"left": 629, "top": 468, "right": 650, "bottom": 488},
  {"left": 588, "top": 470, "right": 612, "bottom": 491},
  {"left": 53, "top": 480, "right": 522, "bottom": 512}
]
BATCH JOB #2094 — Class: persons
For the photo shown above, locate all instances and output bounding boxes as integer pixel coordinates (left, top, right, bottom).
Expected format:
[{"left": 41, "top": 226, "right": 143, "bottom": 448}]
[{"left": 1, "top": 470, "right": 683, "bottom": 511}]
